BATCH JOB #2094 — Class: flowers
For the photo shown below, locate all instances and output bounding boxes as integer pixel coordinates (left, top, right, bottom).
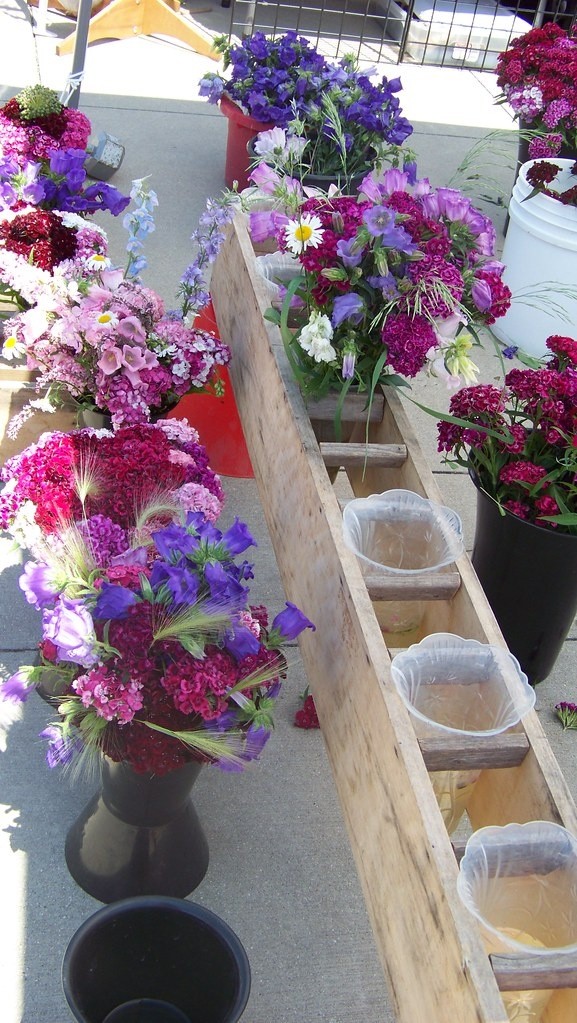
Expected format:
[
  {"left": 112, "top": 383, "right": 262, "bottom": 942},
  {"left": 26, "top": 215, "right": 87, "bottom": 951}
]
[{"left": 0, "top": 20, "right": 577, "bottom": 787}]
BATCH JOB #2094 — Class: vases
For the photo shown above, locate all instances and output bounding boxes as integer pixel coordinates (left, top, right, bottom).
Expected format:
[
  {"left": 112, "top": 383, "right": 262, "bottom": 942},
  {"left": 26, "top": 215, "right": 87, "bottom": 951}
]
[
  {"left": 502, "top": 115, "right": 577, "bottom": 238},
  {"left": 69, "top": 391, "right": 177, "bottom": 432},
  {"left": 246, "top": 135, "right": 379, "bottom": 198},
  {"left": 467, "top": 427, "right": 577, "bottom": 685},
  {"left": 457, "top": 820, "right": 577, "bottom": 1023},
  {"left": 61, "top": 896, "right": 251, "bottom": 1023},
  {"left": 341, "top": 488, "right": 466, "bottom": 648},
  {"left": 389, "top": 632, "right": 537, "bottom": 837},
  {"left": 28, "top": 648, "right": 210, "bottom": 902}
]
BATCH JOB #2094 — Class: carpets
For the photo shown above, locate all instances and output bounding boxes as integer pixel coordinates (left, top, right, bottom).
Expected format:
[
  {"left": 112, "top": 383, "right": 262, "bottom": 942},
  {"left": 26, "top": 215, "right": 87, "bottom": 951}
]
[{"left": 165, "top": 295, "right": 256, "bottom": 479}]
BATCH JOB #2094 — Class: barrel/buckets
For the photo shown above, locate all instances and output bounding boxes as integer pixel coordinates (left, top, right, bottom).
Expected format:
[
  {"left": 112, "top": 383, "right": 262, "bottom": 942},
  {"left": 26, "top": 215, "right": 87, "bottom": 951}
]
[{"left": 481, "top": 154, "right": 577, "bottom": 364}]
